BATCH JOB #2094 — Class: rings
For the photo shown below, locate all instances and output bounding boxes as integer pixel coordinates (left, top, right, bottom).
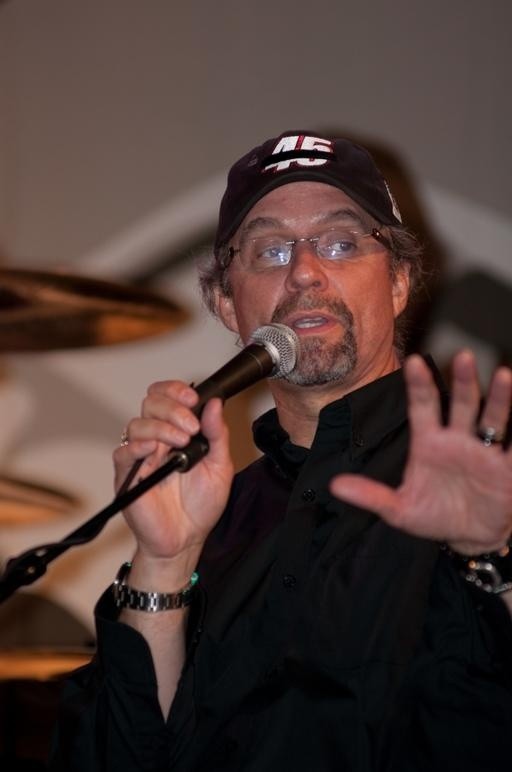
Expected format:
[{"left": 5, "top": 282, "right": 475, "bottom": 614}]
[
  {"left": 478, "top": 431, "right": 504, "bottom": 443},
  {"left": 120, "top": 426, "right": 129, "bottom": 447}
]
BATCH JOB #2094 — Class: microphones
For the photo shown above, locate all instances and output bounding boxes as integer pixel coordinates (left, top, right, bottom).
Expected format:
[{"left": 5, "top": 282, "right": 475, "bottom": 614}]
[{"left": 180, "top": 322, "right": 302, "bottom": 421}]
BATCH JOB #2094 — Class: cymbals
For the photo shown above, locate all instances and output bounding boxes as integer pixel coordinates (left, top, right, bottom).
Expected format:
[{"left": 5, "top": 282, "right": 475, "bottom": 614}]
[
  {"left": 1, "top": 268, "right": 184, "bottom": 352},
  {"left": 0, "top": 477, "right": 77, "bottom": 522}
]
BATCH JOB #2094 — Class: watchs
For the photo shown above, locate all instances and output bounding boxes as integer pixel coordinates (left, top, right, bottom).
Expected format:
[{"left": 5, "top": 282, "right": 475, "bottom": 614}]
[{"left": 112, "top": 562, "right": 201, "bottom": 609}]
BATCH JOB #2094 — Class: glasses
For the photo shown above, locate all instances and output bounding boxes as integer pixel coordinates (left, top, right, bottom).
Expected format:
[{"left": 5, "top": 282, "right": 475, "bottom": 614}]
[{"left": 217, "top": 225, "right": 401, "bottom": 276}]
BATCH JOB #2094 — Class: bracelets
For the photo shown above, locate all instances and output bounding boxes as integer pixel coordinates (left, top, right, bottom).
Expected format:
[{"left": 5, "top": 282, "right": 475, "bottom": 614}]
[{"left": 445, "top": 541, "right": 512, "bottom": 593}]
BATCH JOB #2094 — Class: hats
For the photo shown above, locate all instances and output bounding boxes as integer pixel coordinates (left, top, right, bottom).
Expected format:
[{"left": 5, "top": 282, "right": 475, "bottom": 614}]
[{"left": 213, "top": 128, "right": 405, "bottom": 249}]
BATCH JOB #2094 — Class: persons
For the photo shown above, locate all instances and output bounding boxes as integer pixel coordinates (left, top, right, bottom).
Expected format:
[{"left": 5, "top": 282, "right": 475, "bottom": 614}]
[{"left": 92, "top": 129, "right": 512, "bottom": 772}]
